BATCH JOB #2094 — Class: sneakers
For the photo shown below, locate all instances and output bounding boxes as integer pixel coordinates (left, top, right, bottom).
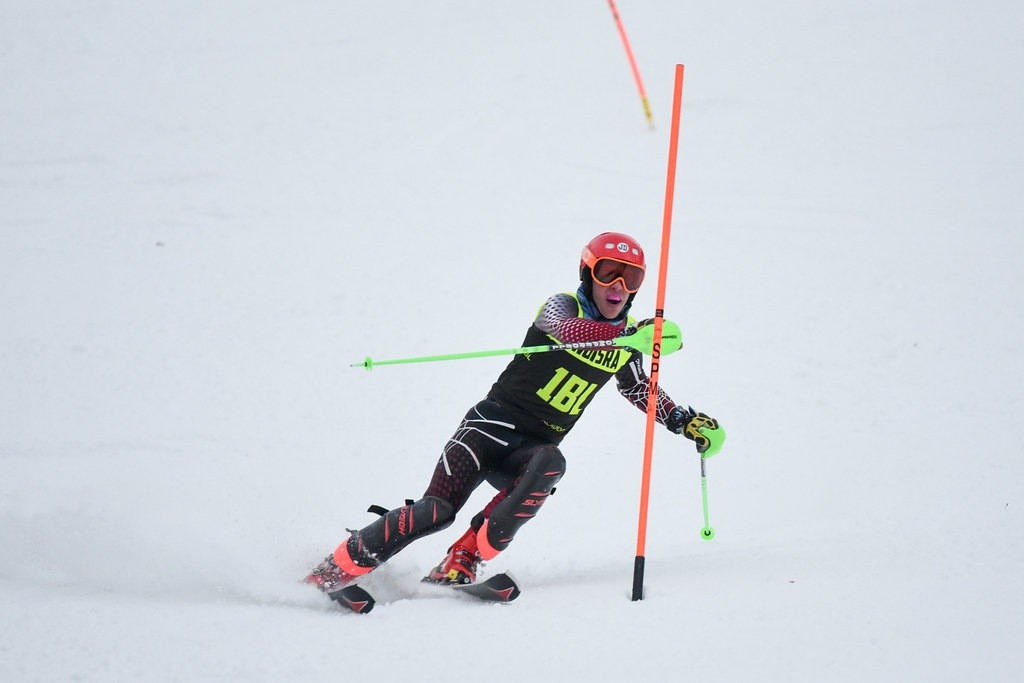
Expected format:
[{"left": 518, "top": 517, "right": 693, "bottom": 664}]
[
  {"left": 435, "top": 549, "right": 477, "bottom": 584},
  {"left": 304, "top": 554, "right": 356, "bottom": 591}
]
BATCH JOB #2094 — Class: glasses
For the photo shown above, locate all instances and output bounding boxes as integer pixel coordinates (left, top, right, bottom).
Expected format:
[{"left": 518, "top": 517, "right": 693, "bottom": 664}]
[{"left": 581, "top": 245, "right": 647, "bottom": 292}]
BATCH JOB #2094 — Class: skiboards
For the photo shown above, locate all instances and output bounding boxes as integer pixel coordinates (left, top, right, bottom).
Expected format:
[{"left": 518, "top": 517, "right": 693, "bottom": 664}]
[
  {"left": 434, "top": 568, "right": 524, "bottom": 604},
  {"left": 330, "top": 582, "right": 376, "bottom": 614}
]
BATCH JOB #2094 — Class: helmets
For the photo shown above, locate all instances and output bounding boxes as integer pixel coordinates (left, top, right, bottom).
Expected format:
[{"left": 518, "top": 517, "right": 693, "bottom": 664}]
[{"left": 579, "top": 232, "right": 646, "bottom": 281}]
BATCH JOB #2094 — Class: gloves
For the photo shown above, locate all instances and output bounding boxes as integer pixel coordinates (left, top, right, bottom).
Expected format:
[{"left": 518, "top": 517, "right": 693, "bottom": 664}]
[
  {"left": 668, "top": 405, "right": 719, "bottom": 453},
  {"left": 620, "top": 317, "right": 683, "bottom": 355}
]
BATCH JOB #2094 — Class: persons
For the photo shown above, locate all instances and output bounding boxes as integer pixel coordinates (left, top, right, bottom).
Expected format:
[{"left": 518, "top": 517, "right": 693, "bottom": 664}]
[{"left": 302, "top": 229, "right": 719, "bottom": 614}]
